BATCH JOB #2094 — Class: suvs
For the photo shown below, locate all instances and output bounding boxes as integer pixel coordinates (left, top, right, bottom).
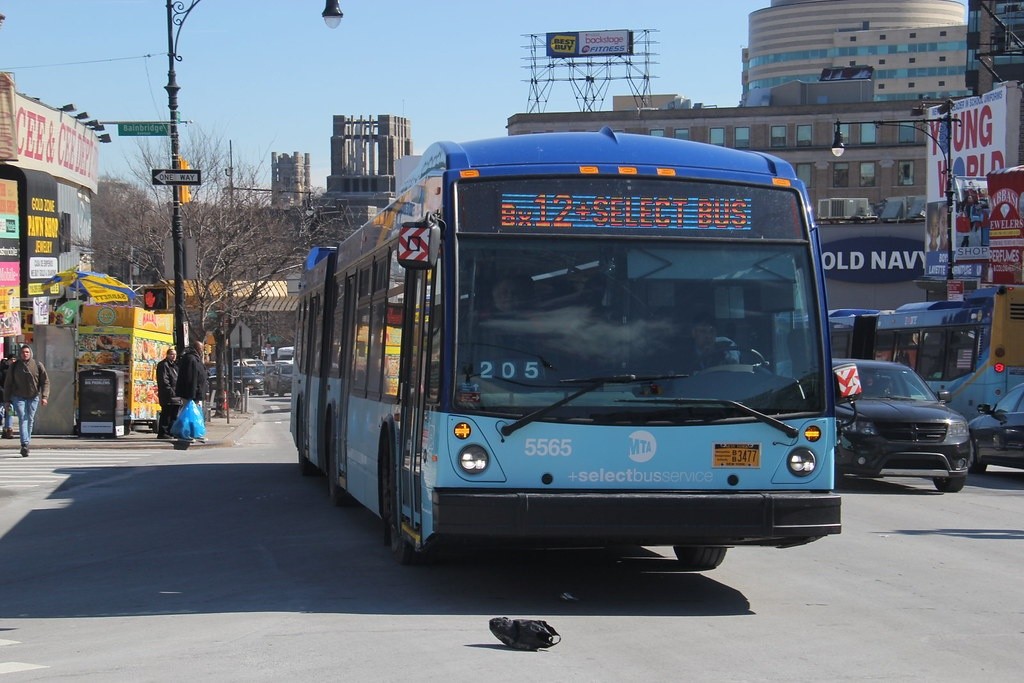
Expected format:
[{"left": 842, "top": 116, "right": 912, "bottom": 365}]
[{"left": 830, "top": 356, "right": 972, "bottom": 492}]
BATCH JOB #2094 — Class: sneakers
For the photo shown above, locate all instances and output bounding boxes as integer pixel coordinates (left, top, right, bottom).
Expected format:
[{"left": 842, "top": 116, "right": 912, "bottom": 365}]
[
  {"left": 178, "top": 437, "right": 195, "bottom": 443},
  {"left": 194, "top": 436, "right": 208, "bottom": 442}
]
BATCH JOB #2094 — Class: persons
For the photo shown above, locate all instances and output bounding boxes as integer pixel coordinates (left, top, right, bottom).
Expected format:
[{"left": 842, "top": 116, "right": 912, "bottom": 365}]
[
  {"left": 0, "top": 354, "right": 18, "bottom": 439},
  {"left": 936, "top": 203, "right": 949, "bottom": 253},
  {"left": 157, "top": 348, "right": 182, "bottom": 439},
  {"left": 667, "top": 314, "right": 740, "bottom": 374},
  {"left": 927, "top": 203, "right": 941, "bottom": 251},
  {"left": 174, "top": 340, "right": 209, "bottom": 444},
  {"left": 959, "top": 194, "right": 978, "bottom": 246},
  {"left": 3, "top": 344, "right": 50, "bottom": 456},
  {"left": 473, "top": 276, "right": 540, "bottom": 323}
]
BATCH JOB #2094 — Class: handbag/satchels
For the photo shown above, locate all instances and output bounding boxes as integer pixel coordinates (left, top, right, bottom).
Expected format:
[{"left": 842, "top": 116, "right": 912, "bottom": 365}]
[{"left": 170, "top": 399, "right": 206, "bottom": 439}]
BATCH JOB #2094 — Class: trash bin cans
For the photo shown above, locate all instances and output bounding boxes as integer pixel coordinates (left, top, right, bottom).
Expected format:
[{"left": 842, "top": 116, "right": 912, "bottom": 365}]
[{"left": 79, "top": 369, "right": 124, "bottom": 439}]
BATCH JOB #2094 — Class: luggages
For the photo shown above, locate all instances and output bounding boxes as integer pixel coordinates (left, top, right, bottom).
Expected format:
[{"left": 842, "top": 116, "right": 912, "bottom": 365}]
[{"left": 21, "top": 444, "right": 28, "bottom": 457}]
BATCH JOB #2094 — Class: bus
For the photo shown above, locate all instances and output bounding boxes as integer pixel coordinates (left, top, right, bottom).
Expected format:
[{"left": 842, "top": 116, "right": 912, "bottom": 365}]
[
  {"left": 291, "top": 126, "right": 847, "bottom": 566},
  {"left": 824, "top": 279, "right": 1023, "bottom": 424}
]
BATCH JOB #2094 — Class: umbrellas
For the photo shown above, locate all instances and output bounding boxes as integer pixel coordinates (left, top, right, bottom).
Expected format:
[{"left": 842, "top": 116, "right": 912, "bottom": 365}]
[{"left": 40, "top": 266, "right": 139, "bottom": 362}]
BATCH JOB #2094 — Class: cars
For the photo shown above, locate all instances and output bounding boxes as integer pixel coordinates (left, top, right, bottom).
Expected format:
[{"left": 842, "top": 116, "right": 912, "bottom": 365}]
[{"left": 965, "top": 381, "right": 1024, "bottom": 474}]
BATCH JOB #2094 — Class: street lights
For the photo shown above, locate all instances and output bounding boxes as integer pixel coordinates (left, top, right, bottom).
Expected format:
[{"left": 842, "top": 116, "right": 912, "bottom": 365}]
[
  {"left": 832, "top": 107, "right": 962, "bottom": 279},
  {"left": 173, "top": 0, "right": 345, "bottom": 366}
]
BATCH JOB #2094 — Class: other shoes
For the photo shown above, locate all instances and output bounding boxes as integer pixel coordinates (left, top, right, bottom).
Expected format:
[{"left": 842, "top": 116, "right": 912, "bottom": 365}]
[
  {"left": 157, "top": 433, "right": 174, "bottom": 439},
  {"left": 168, "top": 434, "right": 179, "bottom": 438}
]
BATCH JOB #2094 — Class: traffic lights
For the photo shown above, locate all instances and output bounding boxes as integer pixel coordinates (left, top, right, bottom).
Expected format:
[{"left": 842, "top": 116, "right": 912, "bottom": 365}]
[{"left": 143, "top": 288, "right": 168, "bottom": 311}]
